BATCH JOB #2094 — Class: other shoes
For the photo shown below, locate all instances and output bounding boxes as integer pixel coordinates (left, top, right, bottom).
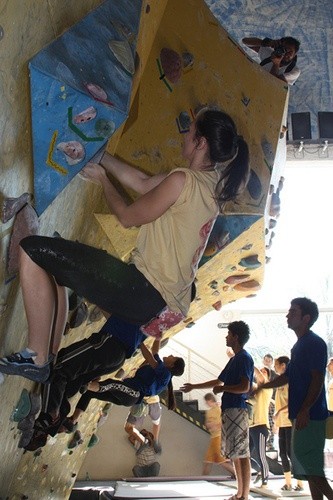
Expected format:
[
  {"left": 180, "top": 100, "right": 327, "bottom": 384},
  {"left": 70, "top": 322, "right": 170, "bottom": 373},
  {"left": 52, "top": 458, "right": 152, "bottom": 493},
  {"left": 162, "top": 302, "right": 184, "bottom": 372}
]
[
  {"left": 281, "top": 484, "right": 292, "bottom": 491},
  {"left": 136, "top": 439, "right": 149, "bottom": 455},
  {"left": 293, "top": 485, "right": 304, "bottom": 491},
  {"left": 152, "top": 441, "right": 162, "bottom": 454},
  {"left": 253, "top": 474, "right": 262, "bottom": 484},
  {"left": 224, "top": 495, "right": 244, "bottom": 500}
]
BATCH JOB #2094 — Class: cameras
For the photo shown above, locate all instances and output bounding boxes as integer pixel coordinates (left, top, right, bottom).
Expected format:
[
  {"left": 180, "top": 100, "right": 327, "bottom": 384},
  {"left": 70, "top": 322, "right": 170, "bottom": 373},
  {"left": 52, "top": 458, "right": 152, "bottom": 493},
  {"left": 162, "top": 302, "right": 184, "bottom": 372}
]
[{"left": 275, "top": 44, "right": 288, "bottom": 57}]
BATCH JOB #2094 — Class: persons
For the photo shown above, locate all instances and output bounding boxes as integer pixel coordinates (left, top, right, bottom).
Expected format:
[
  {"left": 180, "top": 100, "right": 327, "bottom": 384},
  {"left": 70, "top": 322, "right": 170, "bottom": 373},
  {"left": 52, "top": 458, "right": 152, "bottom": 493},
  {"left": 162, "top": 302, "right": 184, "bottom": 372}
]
[
  {"left": 225, "top": 349, "right": 280, "bottom": 486},
  {"left": 125, "top": 394, "right": 162, "bottom": 455},
  {"left": 318, "top": 358, "right": 333, "bottom": 439},
  {"left": 0, "top": 109, "right": 251, "bottom": 385},
  {"left": 272, "top": 356, "right": 305, "bottom": 492},
  {"left": 180, "top": 321, "right": 254, "bottom": 500},
  {"left": 29, "top": 272, "right": 196, "bottom": 431},
  {"left": 57, "top": 331, "right": 185, "bottom": 433},
  {"left": 248, "top": 298, "right": 333, "bottom": 500},
  {"left": 203, "top": 393, "right": 238, "bottom": 476},
  {"left": 242, "top": 36, "right": 301, "bottom": 86},
  {"left": 128, "top": 429, "right": 162, "bottom": 478}
]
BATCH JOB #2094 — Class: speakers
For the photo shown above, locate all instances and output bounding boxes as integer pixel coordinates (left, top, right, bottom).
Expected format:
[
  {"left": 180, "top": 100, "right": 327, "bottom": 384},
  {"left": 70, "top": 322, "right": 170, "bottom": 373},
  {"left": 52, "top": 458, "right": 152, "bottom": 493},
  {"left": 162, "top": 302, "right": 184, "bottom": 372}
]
[
  {"left": 291, "top": 112, "right": 312, "bottom": 140},
  {"left": 318, "top": 112, "right": 333, "bottom": 138}
]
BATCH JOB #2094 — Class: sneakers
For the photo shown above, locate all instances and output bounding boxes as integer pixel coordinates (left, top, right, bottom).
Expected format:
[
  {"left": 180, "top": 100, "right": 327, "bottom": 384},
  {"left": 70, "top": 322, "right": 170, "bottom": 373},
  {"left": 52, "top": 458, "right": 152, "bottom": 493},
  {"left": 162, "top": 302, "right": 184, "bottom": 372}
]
[
  {"left": 0, "top": 350, "right": 50, "bottom": 384},
  {"left": 33, "top": 413, "right": 61, "bottom": 430},
  {"left": 58, "top": 416, "right": 78, "bottom": 434}
]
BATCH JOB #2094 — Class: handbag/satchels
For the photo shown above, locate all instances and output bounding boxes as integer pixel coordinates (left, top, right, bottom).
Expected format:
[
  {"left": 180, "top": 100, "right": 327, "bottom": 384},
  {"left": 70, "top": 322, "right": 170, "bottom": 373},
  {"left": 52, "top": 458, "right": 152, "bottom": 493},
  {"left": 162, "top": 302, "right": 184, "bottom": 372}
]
[{"left": 324, "top": 411, "right": 333, "bottom": 439}]
